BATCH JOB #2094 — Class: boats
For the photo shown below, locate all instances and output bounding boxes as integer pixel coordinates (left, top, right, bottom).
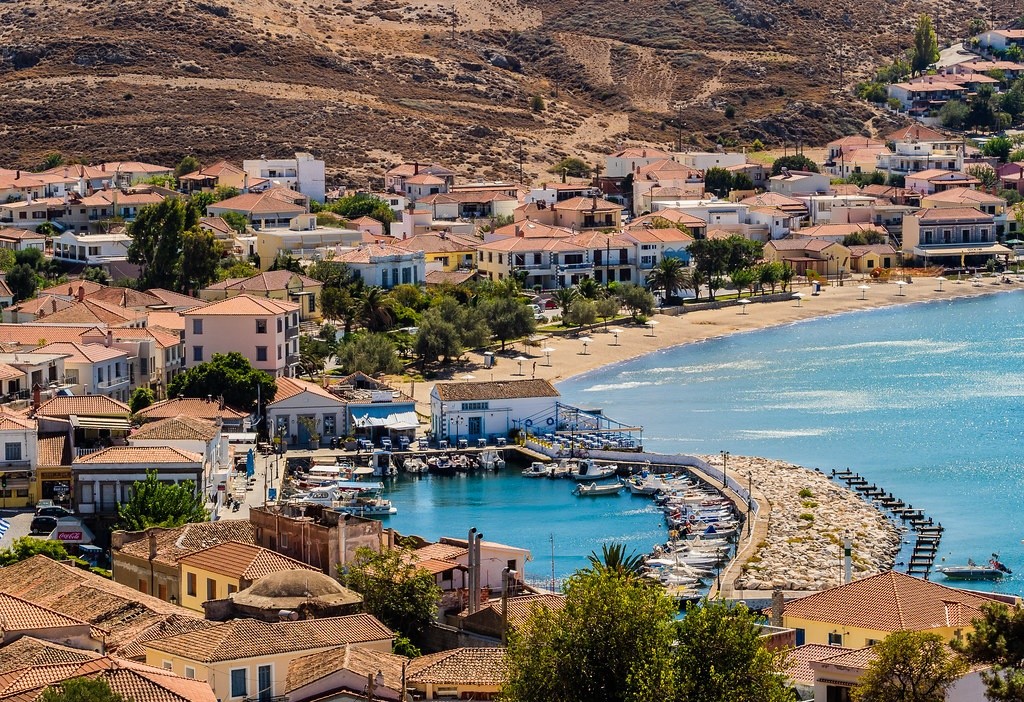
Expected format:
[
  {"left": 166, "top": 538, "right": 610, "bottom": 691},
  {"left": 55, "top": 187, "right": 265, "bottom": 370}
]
[
  {"left": 521, "top": 456, "right": 741, "bottom": 601},
  {"left": 285, "top": 448, "right": 505, "bottom": 515},
  {"left": 934, "top": 550, "right": 1011, "bottom": 580}
]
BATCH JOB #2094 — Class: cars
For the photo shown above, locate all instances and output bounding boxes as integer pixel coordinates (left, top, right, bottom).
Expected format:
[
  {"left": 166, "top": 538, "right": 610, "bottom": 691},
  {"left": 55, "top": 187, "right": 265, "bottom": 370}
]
[
  {"left": 35, "top": 499, "right": 55, "bottom": 516},
  {"left": 527, "top": 304, "right": 543, "bottom": 315},
  {"left": 545, "top": 299, "right": 559, "bottom": 309},
  {"left": 29, "top": 516, "right": 58, "bottom": 536},
  {"left": 36, "top": 504, "right": 75, "bottom": 517},
  {"left": 533, "top": 313, "right": 548, "bottom": 324}
]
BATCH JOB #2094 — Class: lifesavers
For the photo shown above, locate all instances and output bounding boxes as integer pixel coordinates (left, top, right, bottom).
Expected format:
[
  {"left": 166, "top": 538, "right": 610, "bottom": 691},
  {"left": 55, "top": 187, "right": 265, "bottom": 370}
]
[
  {"left": 546, "top": 417, "right": 554, "bottom": 426},
  {"left": 524, "top": 418, "right": 533, "bottom": 428}
]
